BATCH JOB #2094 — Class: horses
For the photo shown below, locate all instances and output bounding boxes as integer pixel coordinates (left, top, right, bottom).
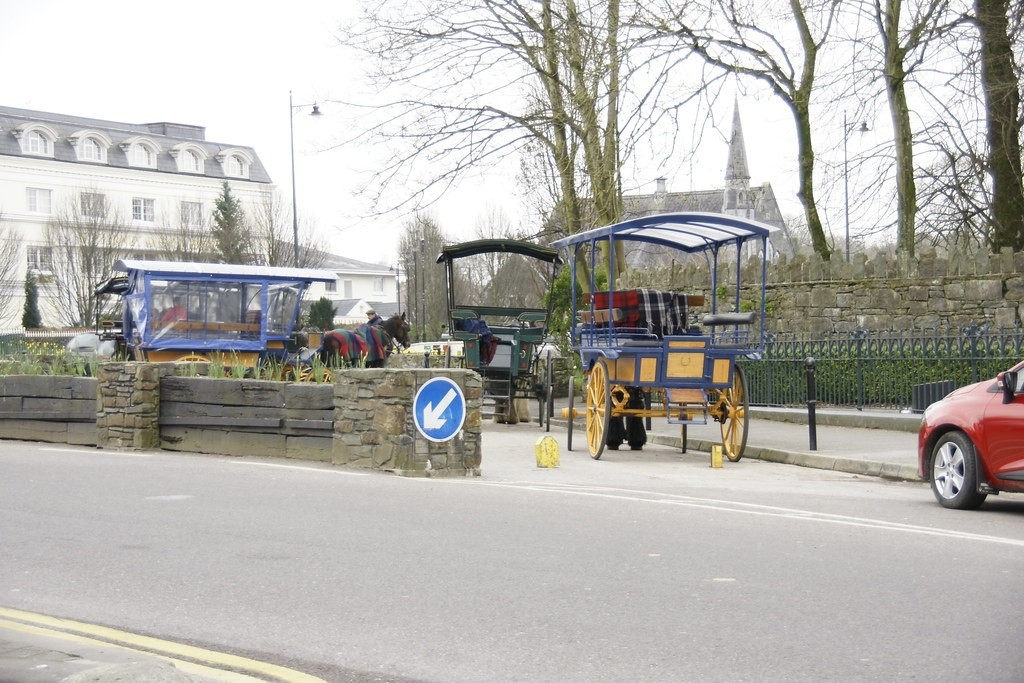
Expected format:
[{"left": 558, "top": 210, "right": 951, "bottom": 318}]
[
  {"left": 606, "top": 386, "right": 647, "bottom": 450},
  {"left": 321, "top": 311, "right": 410, "bottom": 368}
]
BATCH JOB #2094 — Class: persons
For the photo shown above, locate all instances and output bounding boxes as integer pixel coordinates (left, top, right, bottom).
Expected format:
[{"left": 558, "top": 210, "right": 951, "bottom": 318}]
[
  {"left": 607, "top": 391, "right": 647, "bottom": 450},
  {"left": 161, "top": 297, "right": 187, "bottom": 334},
  {"left": 366, "top": 310, "right": 382, "bottom": 324}
]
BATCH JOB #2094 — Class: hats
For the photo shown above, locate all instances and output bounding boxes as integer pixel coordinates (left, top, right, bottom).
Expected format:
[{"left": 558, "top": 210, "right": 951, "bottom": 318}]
[{"left": 366, "top": 310, "right": 375, "bottom": 315}]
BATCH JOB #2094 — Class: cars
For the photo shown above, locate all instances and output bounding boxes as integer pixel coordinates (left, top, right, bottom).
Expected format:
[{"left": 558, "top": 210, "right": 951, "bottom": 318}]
[{"left": 917, "top": 360, "right": 1024, "bottom": 510}]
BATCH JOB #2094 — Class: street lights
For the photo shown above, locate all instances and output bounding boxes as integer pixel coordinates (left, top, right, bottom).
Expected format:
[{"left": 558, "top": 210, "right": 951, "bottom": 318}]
[
  {"left": 843, "top": 109, "right": 871, "bottom": 262},
  {"left": 290, "top": 89, "right": 325, "bottom": 269}
]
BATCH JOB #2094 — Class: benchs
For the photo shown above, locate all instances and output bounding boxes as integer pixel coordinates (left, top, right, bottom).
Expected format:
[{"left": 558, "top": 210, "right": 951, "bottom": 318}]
[
  {"left": 580, "top": 308, "right": 664, "bottom": 347},
  {"left": 451, "top": 309, "right": 491, "bottom": 340},
  {"left": 102, "top": 321, "right": 140, "bottom": 340},
  {"left": 150, "top": 321, "right": 260, "bottom": 341},
  {"left": 703, "top": 313, "right": 755, "bottom": 348},
  {"left": 514, "top": 311, "right": 544, "bottom": 343}
]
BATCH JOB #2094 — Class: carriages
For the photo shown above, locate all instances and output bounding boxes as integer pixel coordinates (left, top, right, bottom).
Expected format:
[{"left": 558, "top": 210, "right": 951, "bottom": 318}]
[
  {"left": 547, "top": 211, "right": 781, "bottom": 459},
  {"left": 434, "top": 239, "right": 566, "bottom": 433},
  {"left": 93, "top": 257, "right": 411, "bottom": 384}
]
